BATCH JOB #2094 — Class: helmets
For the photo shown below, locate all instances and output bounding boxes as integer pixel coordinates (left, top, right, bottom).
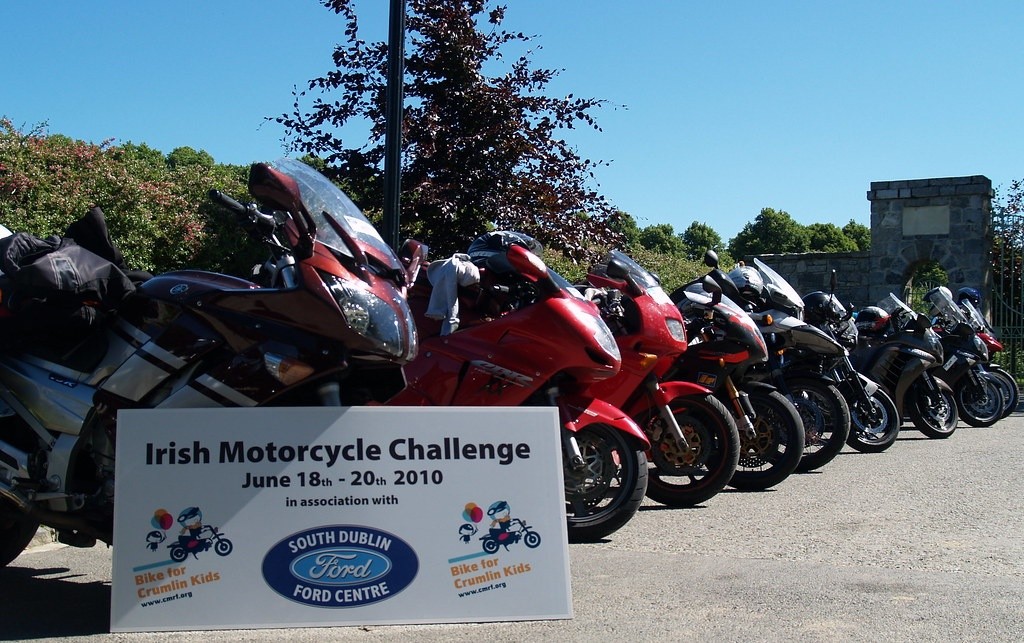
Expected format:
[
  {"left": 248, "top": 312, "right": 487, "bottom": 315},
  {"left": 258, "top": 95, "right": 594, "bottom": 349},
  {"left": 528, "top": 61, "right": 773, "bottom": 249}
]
[
  {"left": 921, "top": 285, "right": 953, "bottom": 317},
  {"left": 956, "top": 286, "right": 980, "bottom": 306},
  {"left": 728, "top": 265, "right": 764, "bottom": 299},
  {"left": 854, "top": 306, "right": 891, "bottom": 341}
]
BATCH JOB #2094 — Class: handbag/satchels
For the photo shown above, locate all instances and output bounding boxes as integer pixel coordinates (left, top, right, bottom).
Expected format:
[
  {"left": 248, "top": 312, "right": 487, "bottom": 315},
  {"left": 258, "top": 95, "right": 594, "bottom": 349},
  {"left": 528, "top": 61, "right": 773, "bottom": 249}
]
[{"left": 1, "top": 207, "right": 139, "bottom": 311}]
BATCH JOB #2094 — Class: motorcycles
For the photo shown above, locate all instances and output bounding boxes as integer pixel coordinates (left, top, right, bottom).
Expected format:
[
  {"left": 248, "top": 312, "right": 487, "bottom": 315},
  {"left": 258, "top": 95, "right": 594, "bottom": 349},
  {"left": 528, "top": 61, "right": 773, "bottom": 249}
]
[
  {"left": 956, "top": 285, "right": 1021, "bottom": 420},
  {"left": 852, "top": 289, "right": 959, "bottom": 438},
  {"left": 921, "top": 285, "right": 1006, "bottom": 428},
  {"left": 726, "top": 256, "right": 851, "bottom": 472},
  {"left": 800, "top": 268, "right": 901, "bottom": 454},
  {"left": 668, "top": 249, "right": 806, "bottom": 491},
  {"left": 572, "top": 247, "right": 741, "bottom": 507},
  {"left": 410, "top": 225, "right": 652, "bottom": 545},
  {"left": 0, "top": 158, "right": 430, "bottom": 568}
]
